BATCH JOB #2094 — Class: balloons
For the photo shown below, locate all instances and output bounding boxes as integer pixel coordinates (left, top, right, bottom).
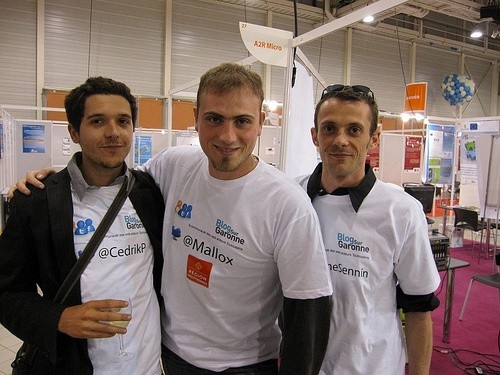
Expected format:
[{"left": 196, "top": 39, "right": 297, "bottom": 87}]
[{"left": 442, "top": 74, "right": 475, "bottom": 105}]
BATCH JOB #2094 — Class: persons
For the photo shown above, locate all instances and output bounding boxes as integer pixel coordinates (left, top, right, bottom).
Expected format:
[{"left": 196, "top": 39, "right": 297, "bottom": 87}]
[{"left": 0, "top": 62, "right": 442, "bottom": 375}]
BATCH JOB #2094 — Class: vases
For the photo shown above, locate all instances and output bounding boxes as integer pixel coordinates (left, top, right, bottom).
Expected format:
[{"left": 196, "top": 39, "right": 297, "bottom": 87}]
[{"left": 456, "top": 103, "right": 463, "bottom": 118}]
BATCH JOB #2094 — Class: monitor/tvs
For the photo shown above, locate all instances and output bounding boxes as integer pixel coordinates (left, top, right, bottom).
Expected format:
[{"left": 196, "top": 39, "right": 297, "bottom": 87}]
[{"left": 404, "top": 185, "right": 435, "bottom": 224}]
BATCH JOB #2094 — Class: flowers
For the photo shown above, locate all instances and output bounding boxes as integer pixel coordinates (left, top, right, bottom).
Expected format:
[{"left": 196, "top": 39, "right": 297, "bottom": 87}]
[{"left": 440, "top": 72, "right": 475, "bottom": 105}]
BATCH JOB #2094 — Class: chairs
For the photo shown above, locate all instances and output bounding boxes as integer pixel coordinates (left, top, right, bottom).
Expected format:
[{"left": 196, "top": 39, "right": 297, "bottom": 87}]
[
  {"left": 453, "top": 207, "right": 486, "bottom": 253},
  {"left": 458, "top": 254, "right": 500, "bottom": 320}
]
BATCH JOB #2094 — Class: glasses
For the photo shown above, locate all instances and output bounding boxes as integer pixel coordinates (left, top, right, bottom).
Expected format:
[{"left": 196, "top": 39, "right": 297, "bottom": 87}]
[{"left": 321, "top": 84, "right": 374, "bottom": 100}]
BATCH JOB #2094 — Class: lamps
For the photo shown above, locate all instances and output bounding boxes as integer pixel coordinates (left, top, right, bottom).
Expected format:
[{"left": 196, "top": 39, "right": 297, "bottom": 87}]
[{"left": 469, "top": 25, "right": 483, "bottom": 38}]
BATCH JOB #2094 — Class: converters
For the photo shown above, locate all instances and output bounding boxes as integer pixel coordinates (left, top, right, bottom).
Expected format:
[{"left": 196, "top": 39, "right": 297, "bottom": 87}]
[{"left": 475, "top": 366, "right": 483, "bottom": 375}]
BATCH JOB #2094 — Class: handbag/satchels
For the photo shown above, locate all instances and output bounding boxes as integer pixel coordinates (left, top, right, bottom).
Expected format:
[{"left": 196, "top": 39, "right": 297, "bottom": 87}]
[{"left": 10, "top": 342, "right": 40, "bottom": 375}]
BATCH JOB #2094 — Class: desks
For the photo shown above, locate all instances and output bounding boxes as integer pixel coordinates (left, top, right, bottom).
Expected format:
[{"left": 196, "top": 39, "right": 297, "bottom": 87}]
[
  {"left": 437, "top": 256, "right": 470, "bottom": 343},
  {"left": 439, "top": 204, "right": 500, "bottom": 220}
]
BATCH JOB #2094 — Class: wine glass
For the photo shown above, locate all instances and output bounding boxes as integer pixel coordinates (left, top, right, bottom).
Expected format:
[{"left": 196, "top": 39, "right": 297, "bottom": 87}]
[{"left": 104, "top": 296, "right": 134, "bottom": 363}]
